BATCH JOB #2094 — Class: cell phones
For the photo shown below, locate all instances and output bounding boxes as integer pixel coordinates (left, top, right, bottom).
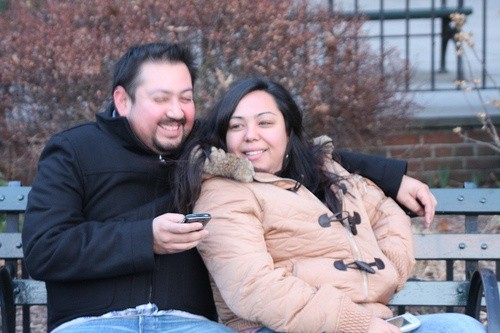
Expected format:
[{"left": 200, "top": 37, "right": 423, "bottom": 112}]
[
  {"left": 384, "top": 312, "right": 422, "bottom": 333},
  {"left": 182, "top": 213, "right": 212, "bottom": 232}
]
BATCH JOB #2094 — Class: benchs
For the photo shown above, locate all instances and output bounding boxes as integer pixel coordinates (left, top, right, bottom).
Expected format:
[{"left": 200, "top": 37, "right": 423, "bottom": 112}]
[{"left": 0, "top": 182, "right": 500, "bottom": 333}]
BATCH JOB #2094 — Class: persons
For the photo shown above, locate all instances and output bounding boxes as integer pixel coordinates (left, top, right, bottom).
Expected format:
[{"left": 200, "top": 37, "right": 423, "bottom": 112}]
[
  {"left": 20, "top": 40, "right": 439, "bottom": 333},
  {"left": 171, "top": 76, "right": 488, "bottom": 333}
]
